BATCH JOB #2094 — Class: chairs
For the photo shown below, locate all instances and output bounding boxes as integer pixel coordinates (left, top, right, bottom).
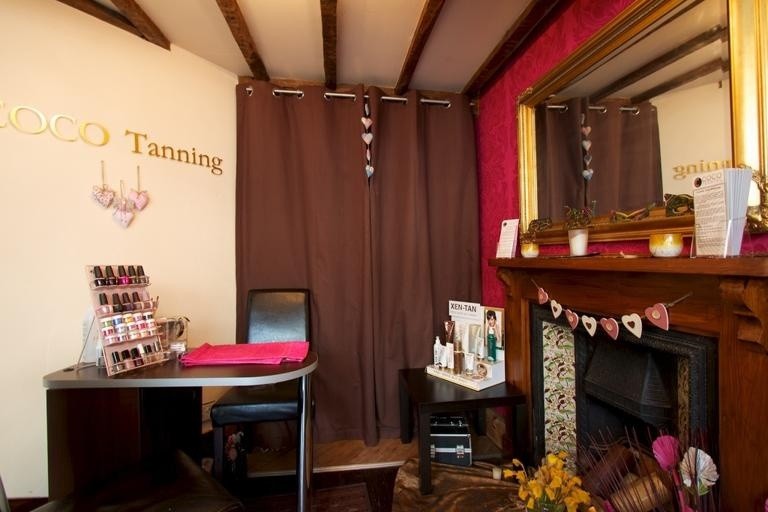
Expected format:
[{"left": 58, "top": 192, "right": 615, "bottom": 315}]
[
  {"left": 0, "top": 447, "right": 244, "bottom": 512},
  {"left": 211, "top": 288, "right": 317, "bottom": 478}
]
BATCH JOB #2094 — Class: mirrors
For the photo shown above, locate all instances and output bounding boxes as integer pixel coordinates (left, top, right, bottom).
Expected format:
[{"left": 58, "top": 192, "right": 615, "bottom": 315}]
[{"left": 515, "top": 0, "right": 768, "bottom": 246}]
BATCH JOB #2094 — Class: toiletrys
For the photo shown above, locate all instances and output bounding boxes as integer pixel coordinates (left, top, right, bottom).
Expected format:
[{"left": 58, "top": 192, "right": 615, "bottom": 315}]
[
  {"left": 94, "top": 265, "right": 165, "bottom": 373},
  {"left": 434, "top": 320, "right": 497, "bottom": 379}
]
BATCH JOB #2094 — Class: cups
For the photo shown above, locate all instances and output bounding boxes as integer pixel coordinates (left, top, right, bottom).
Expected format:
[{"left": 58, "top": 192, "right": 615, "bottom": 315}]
[{"left": 568, "top": 228, "right": 589, "bottom": 256}]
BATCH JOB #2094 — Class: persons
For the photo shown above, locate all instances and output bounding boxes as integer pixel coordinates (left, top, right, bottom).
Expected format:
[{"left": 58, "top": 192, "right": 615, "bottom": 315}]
[{"left": 486, "top": 310, "right": 500, "bottom": 346}]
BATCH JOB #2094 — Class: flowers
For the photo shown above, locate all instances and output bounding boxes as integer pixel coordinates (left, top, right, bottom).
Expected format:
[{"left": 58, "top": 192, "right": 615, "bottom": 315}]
[{"left": 491, "top": 448, "right": 603, "bottom": 512}]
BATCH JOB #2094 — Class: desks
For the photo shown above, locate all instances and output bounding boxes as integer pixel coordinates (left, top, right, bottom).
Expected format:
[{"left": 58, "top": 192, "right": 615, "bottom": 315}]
[
  {"left": 41, "top": 352, "right": 320, "bottom": 512},
  {"left": 399, "top": 369, "right": 529, "bottom": 496}
]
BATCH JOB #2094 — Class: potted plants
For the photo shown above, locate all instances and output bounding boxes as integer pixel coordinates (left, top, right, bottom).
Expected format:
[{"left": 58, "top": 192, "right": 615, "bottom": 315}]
[
  {"left": 562, "top": 201, "right": 591, "bottom": 256},
  {"left": 519, "top": 216, "right": 553, "bottom": 258}
]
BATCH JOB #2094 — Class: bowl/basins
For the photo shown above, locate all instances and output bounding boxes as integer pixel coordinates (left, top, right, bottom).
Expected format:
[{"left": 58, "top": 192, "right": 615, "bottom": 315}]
[{"left": 520, "top": 243, "right": 540, "bottom": 258}]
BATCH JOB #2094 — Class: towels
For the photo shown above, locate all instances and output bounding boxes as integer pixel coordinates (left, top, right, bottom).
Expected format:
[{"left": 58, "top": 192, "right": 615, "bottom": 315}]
[{"left": 180, "top": 340, "right": 312, "bottom": 365}]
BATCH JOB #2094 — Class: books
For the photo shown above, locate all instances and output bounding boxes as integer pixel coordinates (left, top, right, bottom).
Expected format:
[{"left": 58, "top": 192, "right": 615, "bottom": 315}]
[
  {"left": 180, "top": 342, "right": 310, "bottom": 367},
  {"left": 692, "top": 168, "right": 750, "bottom": 257}
]
